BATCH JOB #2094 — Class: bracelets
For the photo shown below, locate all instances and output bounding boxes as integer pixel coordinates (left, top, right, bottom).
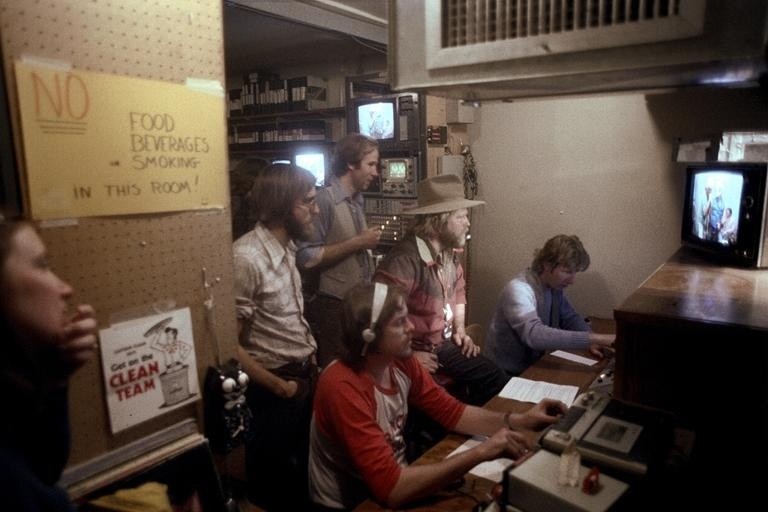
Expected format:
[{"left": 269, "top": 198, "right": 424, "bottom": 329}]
[{"left": 504, "top": 411, "right": 512, "bottom": 429}]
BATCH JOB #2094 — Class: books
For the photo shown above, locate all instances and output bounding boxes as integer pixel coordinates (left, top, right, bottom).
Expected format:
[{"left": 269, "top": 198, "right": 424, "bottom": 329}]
[
  {"left": 227, "top": 119, "right": 323, "bottom": 144},
  {"left": 228, "top": 77, "right": 328, "bottom": 115}
]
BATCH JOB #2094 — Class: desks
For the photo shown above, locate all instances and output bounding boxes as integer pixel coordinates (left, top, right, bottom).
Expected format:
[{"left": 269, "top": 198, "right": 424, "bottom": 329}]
[{"left": 353, "top": 245, "right": 768, "bottom": 512}]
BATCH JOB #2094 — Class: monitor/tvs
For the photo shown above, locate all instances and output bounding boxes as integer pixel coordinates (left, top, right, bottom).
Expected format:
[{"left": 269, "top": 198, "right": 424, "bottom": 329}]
[
  {"left": 378, "top": 157, "right": 418, "bottom": 181},
  {"left": 679, "top": 160, "right": 768, "bottom": 269},
  {"left": 346, "top": 93, "right": 419, "bottom": 154},
  {"left": 291, "top": 147, "right": 330, "bottom": 187}
]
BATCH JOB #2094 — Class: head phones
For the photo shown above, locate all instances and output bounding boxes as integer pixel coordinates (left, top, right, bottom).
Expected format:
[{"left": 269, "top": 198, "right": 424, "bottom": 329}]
[{"left": 357, "top": 282, "right": 390, "bottom": 357}]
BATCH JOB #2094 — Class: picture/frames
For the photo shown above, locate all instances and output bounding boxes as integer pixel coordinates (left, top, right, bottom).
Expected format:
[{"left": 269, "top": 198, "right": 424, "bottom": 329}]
[
  {"left": 12, "top": 57, "right": 227, "bottom": 221},
  {"left": 97, "top": 307, "right": 204, "bottom": 437}
]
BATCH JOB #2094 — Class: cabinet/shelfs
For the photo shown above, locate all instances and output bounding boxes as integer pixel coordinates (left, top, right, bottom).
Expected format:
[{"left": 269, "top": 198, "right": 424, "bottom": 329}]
[
  {"left": 341, "top": 93, "right": 469, "bottom": 270},
  {"left": 227, "top": 105, "right": 347, "bottom": 190}
]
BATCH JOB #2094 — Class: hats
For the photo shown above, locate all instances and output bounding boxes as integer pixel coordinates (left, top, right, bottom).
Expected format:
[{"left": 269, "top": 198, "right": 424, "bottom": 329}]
[{"left": 400, "top": 173, "right": 486, "bottom": 216}]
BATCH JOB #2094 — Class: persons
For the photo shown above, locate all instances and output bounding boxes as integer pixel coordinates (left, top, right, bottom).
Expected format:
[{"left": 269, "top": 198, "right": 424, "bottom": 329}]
[
  {"left": 295, "top": 131, "right": 383, "bottom": 368},
  {"left": 694, "top": 183, "right": 712, "bottom": 240},
  {"left": 718, "top": 208, "right": 736, "bottom": 248},
  {"left": 151, "top": 327, "right": 192, "bottom": 374},
  {"left": 0, "top": 213, "right": 98, "bottom": 512},
  {"left": 706, "top": 192, "right": 726, "bottom": 242},
  {"left": 373, "top": 173, "right": 504, "bottom": 406},
  {"left": 307, "top": 282, "right": 568, "bottom": 512},
  {"left": 231, "top": 162, "right": 319, "bottom": 512},
  {"left": 480, "top": 235, "right": 615, "bottom": 380}
]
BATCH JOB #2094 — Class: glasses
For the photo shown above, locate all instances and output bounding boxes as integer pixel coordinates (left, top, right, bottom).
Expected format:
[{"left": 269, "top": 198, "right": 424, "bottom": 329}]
[{"left": 294, "top": 196, "right": 318, "bottom": 208}]
